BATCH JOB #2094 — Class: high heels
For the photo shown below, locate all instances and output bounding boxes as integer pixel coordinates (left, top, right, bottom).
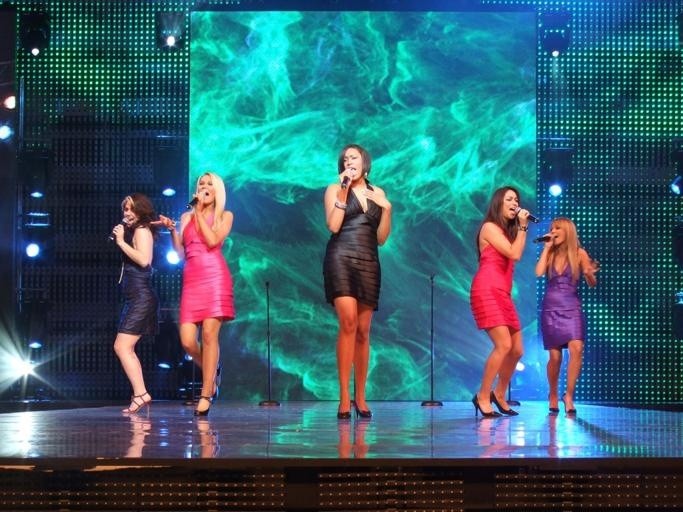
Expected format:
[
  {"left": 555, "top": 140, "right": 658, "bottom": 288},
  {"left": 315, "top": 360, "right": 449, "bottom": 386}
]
[
  {"left": 337, "top": 404, "right": 351, "bottom": 419},
  {"left": 472, "top": 394, "right": 501, "bottom": 417},
  {"left": 490, "top": 390, "right": 519, "bottom": 416},
  {"left": 353, "top": 400, "right": 372, "bottom": 418},
  {"left": 122, "top": 391, "right": 152, "bottom": 414},
  {"left": 548, "top": 392, "right": 560, "bottom": 412},
  {"left": 561, "top": 393, "right": 576, "bottom": 413}
]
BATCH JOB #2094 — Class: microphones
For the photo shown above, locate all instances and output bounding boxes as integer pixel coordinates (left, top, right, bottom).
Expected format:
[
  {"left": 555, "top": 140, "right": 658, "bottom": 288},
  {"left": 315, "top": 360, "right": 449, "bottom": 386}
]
[
  {"left": 533, "top": 236, "right": 551, "bottom": 243},
  {"left": 185, "top": 191, "right": 205, "bottom": 209},
  {"left": 514, "top": 206, "right": 539, "bottom": 224},
  {"left": 340, "top": 168, "right": 354, "bottom": 190},
  {"left": 107, "top": 217, "right": 129, "bottom": 242}
]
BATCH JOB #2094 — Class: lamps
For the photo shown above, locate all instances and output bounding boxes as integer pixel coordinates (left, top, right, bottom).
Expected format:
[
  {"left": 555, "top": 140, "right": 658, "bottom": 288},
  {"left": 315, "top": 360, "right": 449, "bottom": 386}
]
[
  {"left": 24, "top": 161, "right": 48, "bottom": 200},
  {"left": 541, "top": 12, "right": 568, "bottom": 60},
  {"left": 669, "top": 175, "right": 682, "bottom": 195},
  {"left": 541, "top": 143, "right": 573, "bottom": 198},
  {"left": 24, "top": 306, "right": 46, "bottom": 352},
  {"left": 19, "top": 12, "right": 48, "bottom": 58}
]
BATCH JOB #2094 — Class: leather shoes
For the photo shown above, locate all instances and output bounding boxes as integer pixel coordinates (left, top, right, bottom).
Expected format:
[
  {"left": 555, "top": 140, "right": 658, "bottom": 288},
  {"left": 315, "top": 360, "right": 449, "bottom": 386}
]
[{"left": 193, "top": 395, "right": 213, "bottom": 416}]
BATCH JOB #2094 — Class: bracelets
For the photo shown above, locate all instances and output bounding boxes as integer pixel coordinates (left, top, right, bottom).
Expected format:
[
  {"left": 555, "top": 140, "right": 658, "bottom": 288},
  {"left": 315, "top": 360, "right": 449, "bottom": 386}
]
[
  {"left": 168, "top": 221, "right": 176, "bottom": 231},
  {"left": 518, "top": 225, "right": 529, "bottom": 233},
  {"left": 335, "top": 199, "right": 347, "bottom": 211}
]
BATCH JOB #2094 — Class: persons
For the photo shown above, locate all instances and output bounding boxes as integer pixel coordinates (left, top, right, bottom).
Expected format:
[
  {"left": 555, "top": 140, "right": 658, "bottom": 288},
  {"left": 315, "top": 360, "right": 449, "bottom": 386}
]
[
  {"left": 149, "top": 171, "right": 238, "bottom": 416},
  {"left": 535, "top": 217, "right": 600, "bottom": 414},
  {"left": 320, "top": 144, "right": 392, "bottom": 419},
  {"left": 109, "top": 192, "right": 157, "bottom": 413},
  {"left": 469, "top": 184, "right": 531, "bottom": 419}
]
